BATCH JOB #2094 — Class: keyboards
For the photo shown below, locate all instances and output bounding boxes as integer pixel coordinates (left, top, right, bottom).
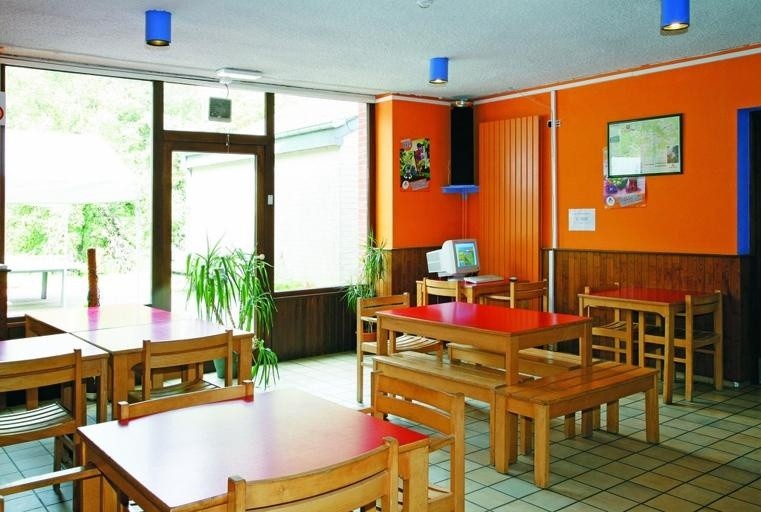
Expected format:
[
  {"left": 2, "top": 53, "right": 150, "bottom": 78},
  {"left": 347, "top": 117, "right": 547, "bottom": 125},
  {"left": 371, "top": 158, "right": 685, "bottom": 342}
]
[{"left": 464, "top": 274, "right": 504, "bottom": 284}]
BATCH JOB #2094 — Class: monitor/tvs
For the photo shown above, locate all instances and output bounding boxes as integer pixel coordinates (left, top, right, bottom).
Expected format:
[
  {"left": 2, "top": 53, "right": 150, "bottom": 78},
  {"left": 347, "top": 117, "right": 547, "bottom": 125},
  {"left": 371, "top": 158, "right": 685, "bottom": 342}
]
[{"left": 426, "top": 238, "right": 480, "bottom": 281}]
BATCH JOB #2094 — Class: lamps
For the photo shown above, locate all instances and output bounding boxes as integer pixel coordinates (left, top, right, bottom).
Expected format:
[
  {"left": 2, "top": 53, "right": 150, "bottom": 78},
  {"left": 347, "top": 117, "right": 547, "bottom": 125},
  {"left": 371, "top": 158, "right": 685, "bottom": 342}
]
[
  {"left": 145, "top": 10, "right": 172, "bottom": 46},
  {"left": 428, "top": 57, "right": 448, "bottom": 83},
  {"left": 660, "top": 0, "right": 690, "bottom": 31}
]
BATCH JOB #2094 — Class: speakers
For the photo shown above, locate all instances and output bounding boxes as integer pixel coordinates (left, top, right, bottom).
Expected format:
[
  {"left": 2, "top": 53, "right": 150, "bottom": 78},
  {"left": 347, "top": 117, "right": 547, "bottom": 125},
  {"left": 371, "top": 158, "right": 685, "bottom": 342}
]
[{"left": 450, "top": 106, "right": 479, "bottom": 185}]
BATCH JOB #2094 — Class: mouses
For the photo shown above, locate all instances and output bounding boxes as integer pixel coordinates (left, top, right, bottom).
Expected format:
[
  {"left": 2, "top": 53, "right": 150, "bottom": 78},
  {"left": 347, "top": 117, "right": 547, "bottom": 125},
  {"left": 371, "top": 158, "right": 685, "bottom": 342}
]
[{"left": 509, "top": 277, "right": 517, "bottom": 282}]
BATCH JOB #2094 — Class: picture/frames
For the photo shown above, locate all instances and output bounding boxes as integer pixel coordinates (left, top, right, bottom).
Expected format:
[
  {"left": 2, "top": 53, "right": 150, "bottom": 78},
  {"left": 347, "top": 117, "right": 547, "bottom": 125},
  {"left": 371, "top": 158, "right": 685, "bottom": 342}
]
[{"left": 608, "top": 114, "right": 684, "bottom": 178}]
